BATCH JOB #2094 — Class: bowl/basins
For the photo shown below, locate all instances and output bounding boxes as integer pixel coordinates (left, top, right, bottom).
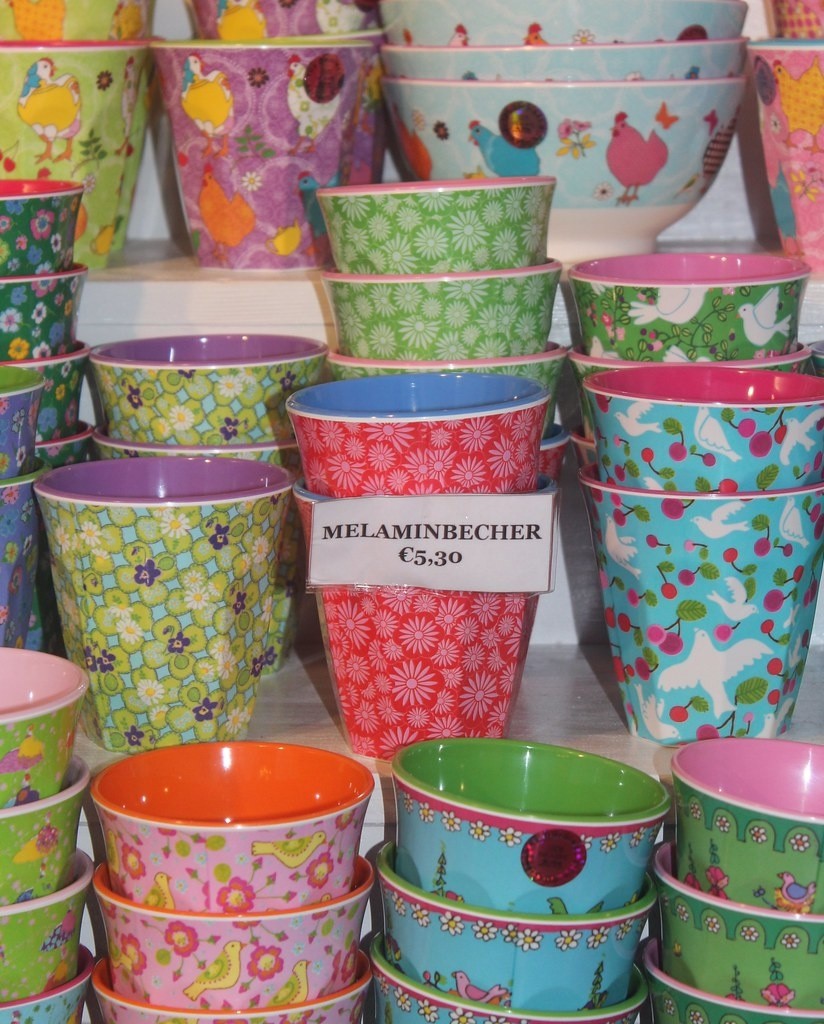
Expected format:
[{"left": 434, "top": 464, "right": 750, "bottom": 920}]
[
  {"left": 377, "top": 1, "right": 749, "bottom": 51},
  {"left": 376, "top": 76, "right": 749, "bottom": 267},
  {"left": 379, "top": 36, "right": 753, "bottom": 81},
  {"left": 0, "top": 648, "right": 823, "bottom": 1024}
]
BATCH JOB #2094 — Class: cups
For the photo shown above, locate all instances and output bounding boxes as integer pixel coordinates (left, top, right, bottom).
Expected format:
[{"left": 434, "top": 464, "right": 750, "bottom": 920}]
[{"left": 0, "top": 2, "right": 824, "bottom": 743}]
